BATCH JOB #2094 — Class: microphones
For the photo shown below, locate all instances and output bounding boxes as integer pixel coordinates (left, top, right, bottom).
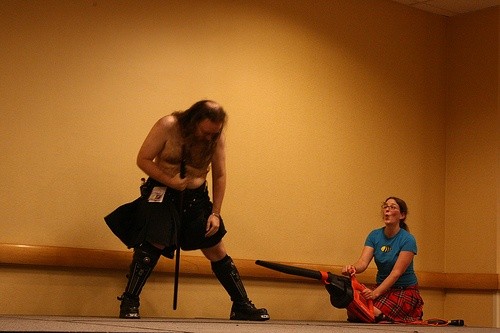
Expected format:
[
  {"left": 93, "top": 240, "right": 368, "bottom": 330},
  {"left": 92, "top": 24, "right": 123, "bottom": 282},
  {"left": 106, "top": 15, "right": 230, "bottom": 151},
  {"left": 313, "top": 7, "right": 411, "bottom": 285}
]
[{"left": 427, "top": 319, "right": 464, "bottom": 326}]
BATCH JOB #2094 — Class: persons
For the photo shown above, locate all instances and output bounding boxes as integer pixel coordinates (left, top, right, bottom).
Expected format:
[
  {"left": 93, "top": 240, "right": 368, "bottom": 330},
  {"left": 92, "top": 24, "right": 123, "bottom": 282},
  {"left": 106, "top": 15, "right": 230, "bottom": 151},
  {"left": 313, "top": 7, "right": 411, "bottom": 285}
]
[
  {"left": 116, "top": 99, "right": 271, "bottom": 321},
  {"left": 341, "top": 196, "right": 425, "bottom": 324}
]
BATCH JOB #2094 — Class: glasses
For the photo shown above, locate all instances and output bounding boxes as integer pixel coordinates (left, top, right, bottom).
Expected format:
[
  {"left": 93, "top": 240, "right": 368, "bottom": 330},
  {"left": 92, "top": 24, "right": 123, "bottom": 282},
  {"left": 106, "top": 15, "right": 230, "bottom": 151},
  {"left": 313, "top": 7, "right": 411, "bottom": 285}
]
[{"left": 381, "top": 204, "right": 401, "bottom": 210}]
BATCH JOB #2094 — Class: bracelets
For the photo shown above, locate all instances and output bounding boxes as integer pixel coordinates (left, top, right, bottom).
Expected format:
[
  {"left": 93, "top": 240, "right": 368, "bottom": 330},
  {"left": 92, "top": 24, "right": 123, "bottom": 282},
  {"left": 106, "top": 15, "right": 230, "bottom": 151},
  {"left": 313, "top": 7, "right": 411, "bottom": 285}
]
[{"left": 211, "top": 212, "right": 221, "bottom": 218}]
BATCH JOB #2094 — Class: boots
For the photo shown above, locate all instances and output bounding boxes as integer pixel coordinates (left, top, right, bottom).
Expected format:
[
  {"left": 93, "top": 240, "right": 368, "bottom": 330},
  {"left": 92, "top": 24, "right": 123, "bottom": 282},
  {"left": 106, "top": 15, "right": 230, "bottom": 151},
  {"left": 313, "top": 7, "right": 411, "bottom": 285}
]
[
  {"left": 117, "top": 248, "right": 160, "bottom": 319},
  {"left": 211, "top": 254, "right": 270, "bottom": 321}
]
